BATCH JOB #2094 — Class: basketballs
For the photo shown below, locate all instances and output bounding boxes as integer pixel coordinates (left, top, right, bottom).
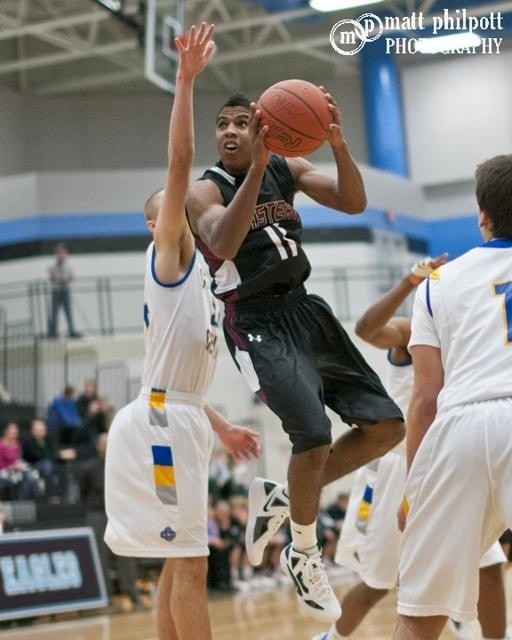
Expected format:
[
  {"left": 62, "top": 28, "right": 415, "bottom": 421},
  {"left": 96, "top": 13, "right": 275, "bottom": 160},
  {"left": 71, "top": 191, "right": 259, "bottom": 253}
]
[{"left": 253, "top": 78, "right": 331, "bottom": 157}]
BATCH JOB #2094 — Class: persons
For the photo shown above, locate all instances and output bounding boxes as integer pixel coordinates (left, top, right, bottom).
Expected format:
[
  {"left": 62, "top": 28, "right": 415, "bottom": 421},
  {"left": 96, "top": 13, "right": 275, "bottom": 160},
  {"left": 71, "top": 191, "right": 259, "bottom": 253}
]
[
  {"left": 104, "top": 21, "right": 512, "bottom": 640},
  {"left": 68, "top": 432, "right": 150, "bottom": 612},
  {"left": 45, "top": 241, "right": 82, "bottom": 339},
  {"left": 0, "top": 380, "right": 104, "bottom": 513}
]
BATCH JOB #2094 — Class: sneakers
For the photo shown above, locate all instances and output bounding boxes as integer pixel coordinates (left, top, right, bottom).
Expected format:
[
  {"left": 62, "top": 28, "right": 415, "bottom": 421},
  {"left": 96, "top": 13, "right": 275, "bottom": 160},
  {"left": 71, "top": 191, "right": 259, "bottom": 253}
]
[
  {"left": 279, "top": 542, "right": 343, "bottom": 623},
  {"left": 244, "top": 476, "right": 290, "bottom": 567}
]
